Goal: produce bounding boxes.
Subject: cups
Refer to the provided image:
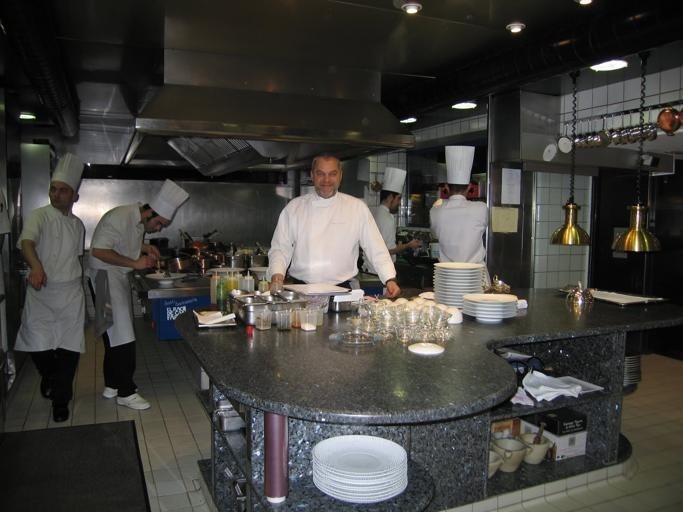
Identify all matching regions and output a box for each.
[522,433,553,465]
[345,298,452,344]
[490,437,532,473]
[487,450,505,479]
[254,298,327,332]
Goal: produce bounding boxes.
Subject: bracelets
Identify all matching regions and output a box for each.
[387,278,400,284]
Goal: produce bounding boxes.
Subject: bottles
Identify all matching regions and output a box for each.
[258,276,268,293]
[209,269,254,311]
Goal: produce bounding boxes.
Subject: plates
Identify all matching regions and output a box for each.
[195,310,237,328]
[433,259,485,308]
[312,434,409,503]
[281,284,351,295]
[461,294,519,325]
[145,273,187,284]
[623,348,642,387]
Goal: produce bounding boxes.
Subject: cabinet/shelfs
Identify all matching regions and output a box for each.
[246,408,493,512]
[488,327,634,512]
[208,384,247,512]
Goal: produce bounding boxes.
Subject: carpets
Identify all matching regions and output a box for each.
[0,420,151,512]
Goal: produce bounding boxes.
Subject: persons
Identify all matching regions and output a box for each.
[429,145,492,287]
[17,152,86,423]
[266,150,402,298]
[362,166,423,275]
[85,179,190,410]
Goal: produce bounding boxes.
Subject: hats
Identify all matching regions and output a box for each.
[444,144,476,186]
[148,177,190,222]
[48,151,85,192]
[381,165,408,195]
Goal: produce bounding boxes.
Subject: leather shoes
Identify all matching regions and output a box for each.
[40,375,56,399]
[52,403,69,421]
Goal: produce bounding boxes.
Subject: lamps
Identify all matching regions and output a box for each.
[610,0,659,251]
[549,0,593,247]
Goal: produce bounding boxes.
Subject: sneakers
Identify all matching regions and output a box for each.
[116,391,152,410]
[102,386,138,399]
